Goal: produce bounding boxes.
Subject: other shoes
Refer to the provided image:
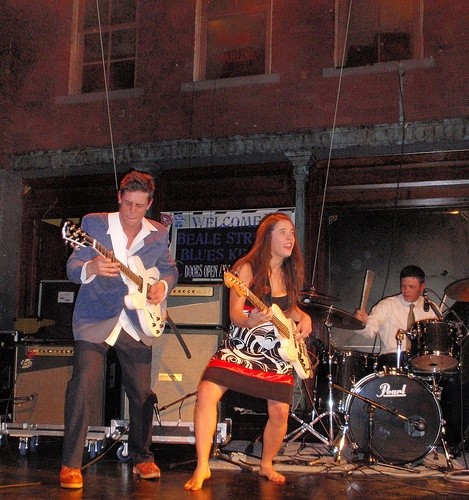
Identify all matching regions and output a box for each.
[59,465,84,488]
[133,462,161,478]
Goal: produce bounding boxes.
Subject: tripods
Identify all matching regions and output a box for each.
[283,321,344,456]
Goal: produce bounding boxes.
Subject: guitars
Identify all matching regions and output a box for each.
[223,262,312,381]
[61,221,168,339]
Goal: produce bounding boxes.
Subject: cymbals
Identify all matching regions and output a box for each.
[299,290,341,302]
[299,302,366,330]
[446,279,469,302]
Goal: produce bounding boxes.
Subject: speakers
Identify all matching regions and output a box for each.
[11,339,105,434]
[120,328,226,427]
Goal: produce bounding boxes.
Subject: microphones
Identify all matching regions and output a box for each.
[414,419,428,431]
[422,290,429,312]
[150,393,162,424]
[27,391,37,402]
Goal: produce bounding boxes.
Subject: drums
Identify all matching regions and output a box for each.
[405,318,459,371]
[314,349,378,411]
[343,370,443,464]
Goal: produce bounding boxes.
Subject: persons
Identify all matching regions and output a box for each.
[353,265,443,372]
[184,213,313,492]
[58,171,179,489]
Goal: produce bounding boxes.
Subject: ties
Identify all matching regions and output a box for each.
[406,304,415,351]
